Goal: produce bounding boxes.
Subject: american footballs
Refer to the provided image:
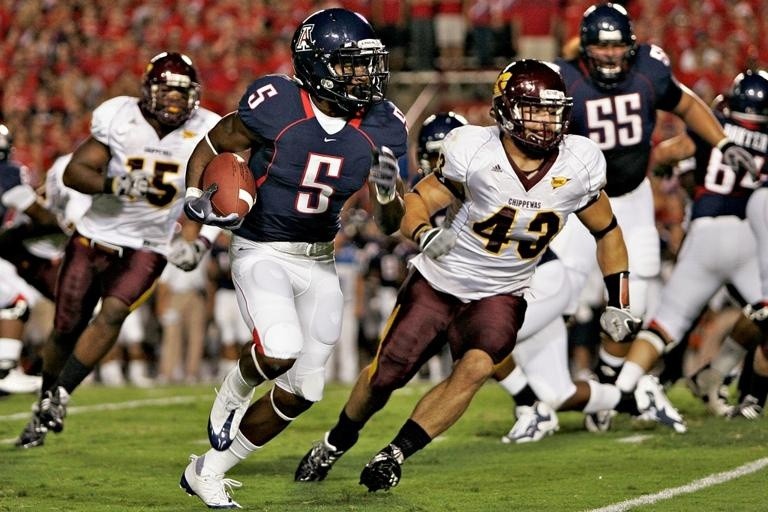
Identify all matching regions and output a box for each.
[203,152,256,222]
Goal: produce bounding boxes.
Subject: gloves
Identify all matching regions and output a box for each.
[101,169,152,199]
[601,308,644,344]
[368,144,400,205]
[716,139,759,184]
[419,227,459,261]
[166,240,206,272]
[183,183,245,231]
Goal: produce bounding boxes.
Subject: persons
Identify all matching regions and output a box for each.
[179,7,407,507]
[340,1,768,258]
[324,229,365,387]
[582,69,768,434]
[15,52,222,446]
[550,0,759,386]
[0,0,319,392]
[293,57,642,492]
[415,111,687,442]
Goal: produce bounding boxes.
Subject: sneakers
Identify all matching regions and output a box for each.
[358,449,400,492]
[15,422,47,449]
[501,370,763,442]
[180,456,242,509]
[208,372,256,452]
[294,428,358,482]
[32,386,67,433]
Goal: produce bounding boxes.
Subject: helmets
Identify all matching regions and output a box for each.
[290,9,390,119]
[139,51,201,127]
[728,69,768,123]
[416,113,469,181]
[581,3,636,91]
[492,59,574,156]
